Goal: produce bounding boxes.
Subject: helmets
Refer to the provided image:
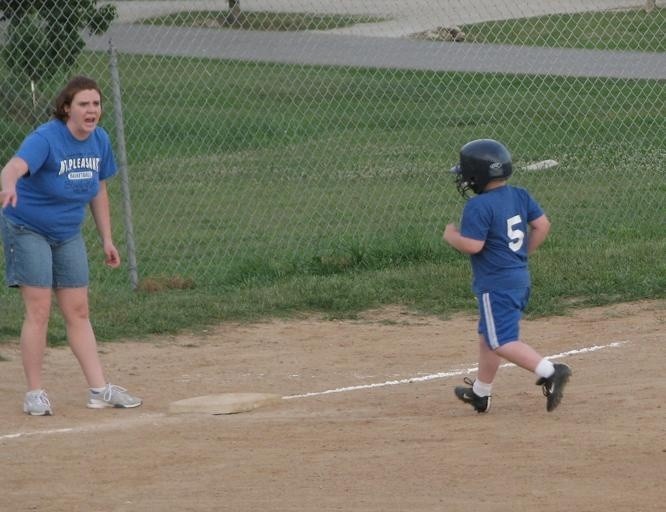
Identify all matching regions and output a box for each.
[450,139,512,199]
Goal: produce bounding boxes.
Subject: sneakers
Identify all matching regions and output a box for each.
[537,363,571,411]
[24,389,52,415]
[455,377,491,413]
[87,384,142,408]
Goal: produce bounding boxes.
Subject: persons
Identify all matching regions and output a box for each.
[0,76,143,417]
[442,138,572,412]
